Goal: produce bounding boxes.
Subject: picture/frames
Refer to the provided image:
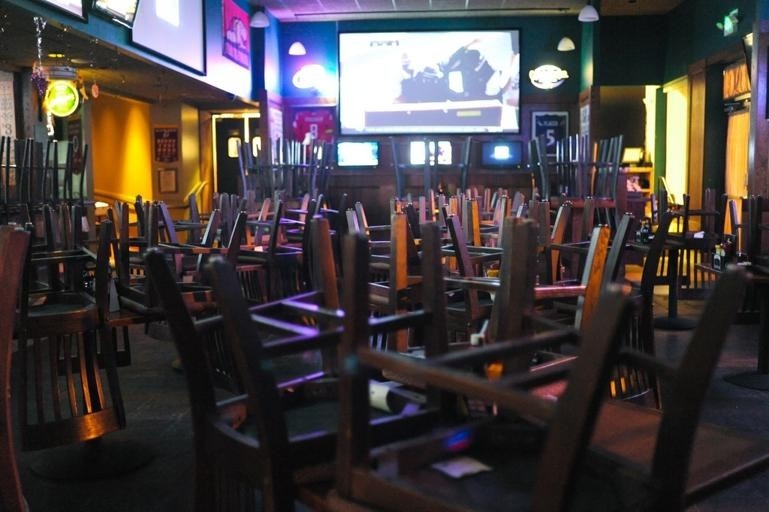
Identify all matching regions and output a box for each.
[157,167,178,193]
[529,109,571,159]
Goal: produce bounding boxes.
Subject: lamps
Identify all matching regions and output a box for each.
[249,11,269,29]
[577,0,599,23]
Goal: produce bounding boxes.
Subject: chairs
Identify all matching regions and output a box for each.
[1,135,768,509]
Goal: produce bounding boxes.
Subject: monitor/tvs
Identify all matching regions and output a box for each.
[480,141,523,170]
[43,0,89,23]
[91,0,139,29]
[336,141,380,169]
[408,140,455,168]
